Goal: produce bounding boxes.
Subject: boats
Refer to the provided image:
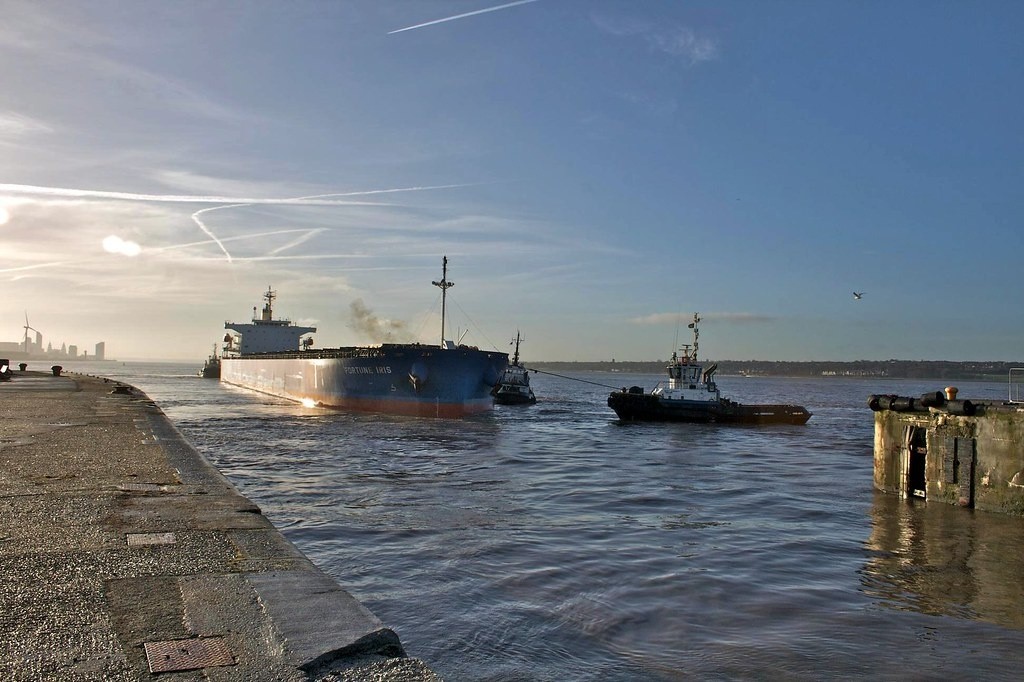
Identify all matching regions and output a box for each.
[608,312,812,425]
[494,330,537,406]
[221,254,512,421]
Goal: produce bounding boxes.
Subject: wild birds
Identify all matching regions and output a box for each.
[852,291,867,301]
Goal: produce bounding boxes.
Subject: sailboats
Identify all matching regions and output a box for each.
[199,342,220,378]
